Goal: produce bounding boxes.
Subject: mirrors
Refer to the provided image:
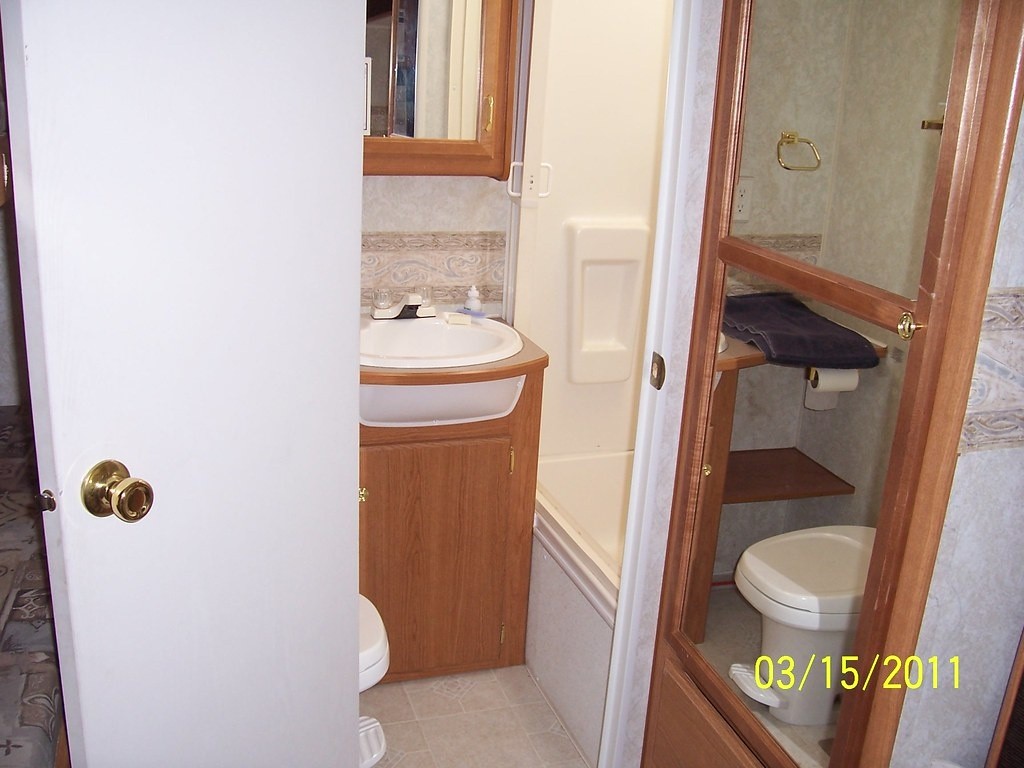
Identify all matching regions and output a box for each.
[666,0,996,768]
[364,0,513,182]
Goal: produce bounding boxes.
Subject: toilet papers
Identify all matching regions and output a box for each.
[803,367,859,411]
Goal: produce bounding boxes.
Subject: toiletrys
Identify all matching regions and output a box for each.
[464,284,482,312]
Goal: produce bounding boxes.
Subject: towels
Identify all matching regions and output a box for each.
[721,291,880,370]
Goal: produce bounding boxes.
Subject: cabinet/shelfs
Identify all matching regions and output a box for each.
[683,322,889,645]
[358,438,514,684]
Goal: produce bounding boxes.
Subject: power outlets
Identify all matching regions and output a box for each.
[731,175,754,223]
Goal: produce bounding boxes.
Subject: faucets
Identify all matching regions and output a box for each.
[369,283,437,320]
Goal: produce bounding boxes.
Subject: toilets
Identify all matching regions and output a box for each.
[728,524,877,724]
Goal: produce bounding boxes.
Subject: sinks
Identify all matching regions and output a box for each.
[359,311,524,369]
